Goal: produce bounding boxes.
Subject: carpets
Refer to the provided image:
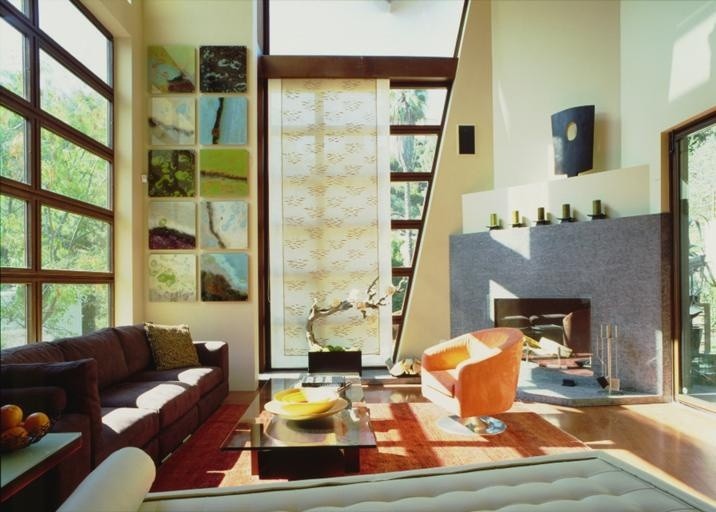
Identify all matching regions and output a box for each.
[148,386,590,493]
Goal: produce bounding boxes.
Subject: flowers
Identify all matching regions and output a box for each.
[307,275,406,352]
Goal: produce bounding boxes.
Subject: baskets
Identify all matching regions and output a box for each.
[0,418,57,451]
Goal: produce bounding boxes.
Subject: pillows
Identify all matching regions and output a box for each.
[144,322,202,371]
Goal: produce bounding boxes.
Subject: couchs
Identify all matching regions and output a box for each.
[0,322,229,511]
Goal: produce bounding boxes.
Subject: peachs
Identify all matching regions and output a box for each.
[1,405,49,440]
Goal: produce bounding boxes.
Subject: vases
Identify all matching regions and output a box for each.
[308,351,362,377]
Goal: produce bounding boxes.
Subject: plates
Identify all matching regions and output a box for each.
[263,396,348,422]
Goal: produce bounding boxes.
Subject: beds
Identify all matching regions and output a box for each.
[57,448,715,511]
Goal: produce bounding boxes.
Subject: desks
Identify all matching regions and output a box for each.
[0,430,83,505]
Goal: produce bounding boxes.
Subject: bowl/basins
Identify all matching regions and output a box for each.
[273,387,340,416]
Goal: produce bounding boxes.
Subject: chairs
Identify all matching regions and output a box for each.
[420,326,524,436]
